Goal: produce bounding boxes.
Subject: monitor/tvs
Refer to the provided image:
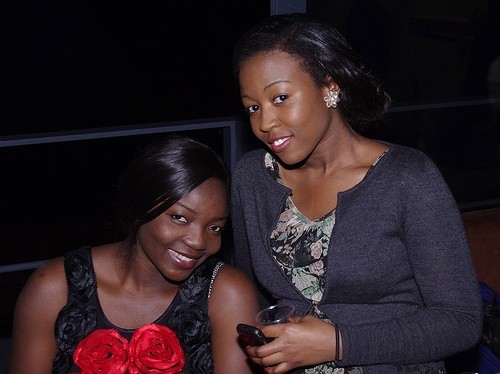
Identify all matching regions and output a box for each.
[351,97,500,212]
[0,117,240,286]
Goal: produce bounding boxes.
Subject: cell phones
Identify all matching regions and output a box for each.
[236,324,268,347]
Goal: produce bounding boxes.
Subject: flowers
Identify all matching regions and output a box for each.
[73,324,185,374]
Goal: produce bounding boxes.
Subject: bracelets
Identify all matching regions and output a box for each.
[336,328,339,360]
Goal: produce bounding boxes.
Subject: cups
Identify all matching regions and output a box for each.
[255,303,297,325]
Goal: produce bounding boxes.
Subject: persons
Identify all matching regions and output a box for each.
[229,12,481,374]
[8,136,260,374]
